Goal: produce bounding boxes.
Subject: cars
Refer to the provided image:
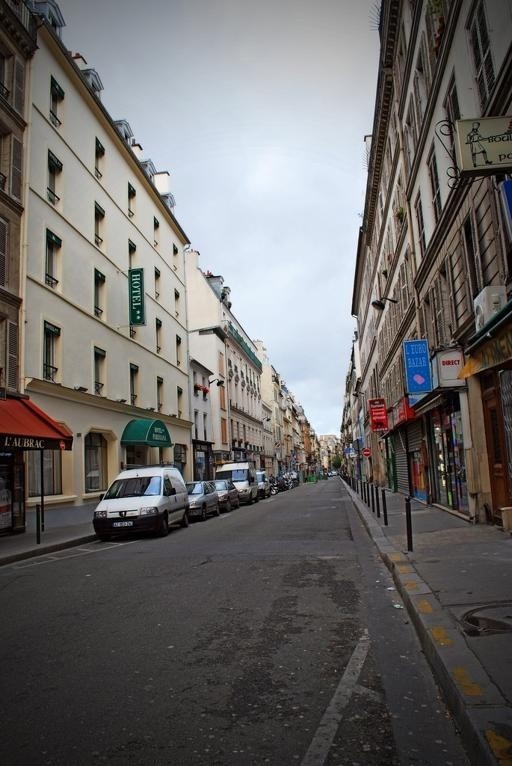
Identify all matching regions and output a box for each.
[185,479,240,522]
[290,471,302,486]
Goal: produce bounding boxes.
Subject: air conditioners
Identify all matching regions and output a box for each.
[472,284,508,333]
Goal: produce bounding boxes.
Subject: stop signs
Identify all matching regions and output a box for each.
[362,448,372,458]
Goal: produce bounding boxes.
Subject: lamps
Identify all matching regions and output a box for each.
[371,296,398,311]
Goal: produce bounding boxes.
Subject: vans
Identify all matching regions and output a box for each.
[215,461,271,504]
[93,466,190,541]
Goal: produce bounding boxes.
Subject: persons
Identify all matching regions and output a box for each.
[311,459,317,474]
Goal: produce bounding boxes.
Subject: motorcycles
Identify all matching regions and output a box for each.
[271,473,293,494]
